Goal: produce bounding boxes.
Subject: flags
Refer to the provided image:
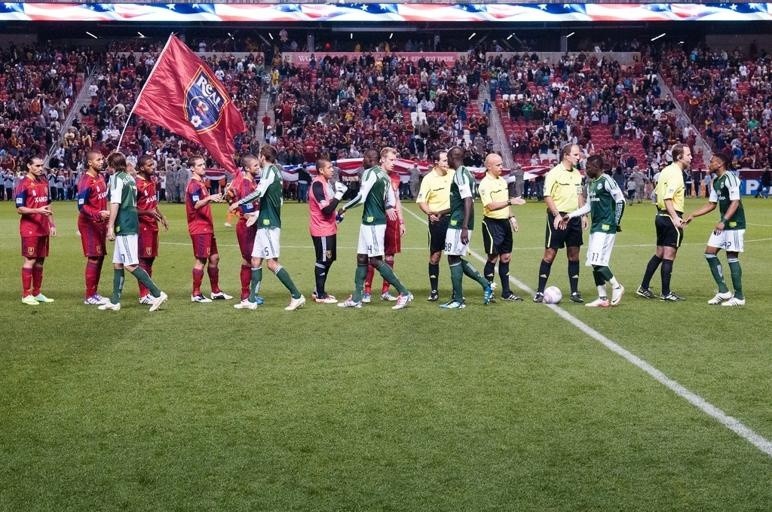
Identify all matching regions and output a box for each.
[116,32,249,174]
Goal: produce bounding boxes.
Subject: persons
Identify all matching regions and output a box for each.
[43,167,82,199]
[683,47,745,153]
[236,155,265,306]
[635,143,685,302]
[229,143,306,312]
[1,41,14,147]
[250,29,306,140]
[56,39,115,150]
[415,150,456,303]
[591,46,634,154]
[59,150,75,200]
[131,155,168,305]
[495,32,532,150]
[607,157,665,202]
[534,44,591,142]
[15,155,59,305]
[635,44,683,143]
[187,155,233,304]
[359,149,406,302]
[532,143,589,302]
[0,150,14,199]
[559,155,626,308]
[746,46,772,169]
[336,148,414,311]
[309,159,345,305]
[479,153,528,304]
[307,30,333,158]
[14,46,56,154]
[685,155,745,307]
[76,151,110,305]
[98,152,168,311]
[335,36,493,148]
[440,145,497,309]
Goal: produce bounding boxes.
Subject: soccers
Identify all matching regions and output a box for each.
[543,286,562,304]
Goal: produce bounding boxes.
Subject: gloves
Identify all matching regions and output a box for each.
[334,209,345,226]
[332,179,348,201]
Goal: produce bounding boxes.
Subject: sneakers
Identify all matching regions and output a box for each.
[148,290,168,312]
[315,296,338,304]
[390,291,415,311]
[35,292,54,302]
[312,292,335,300]
[635,284,658,300]
[337,294,365,309]
[21,293,40,305]
[84,294,109,304]
[610,283,625,306]
[380,291,398,301]
[361,293,372,304]
[482,281,497,306]
[439,297,467,311]
[190,293,213,303]
[500,291,523,302]
[584,297,609,308]
[659,291,686,302]
[706,290,734,305]
[138,293,156,305]
[426,289,439,302]
[233,297,259,312]
[569,292,584,304]
[256,296,264,305]
[720,295,747,308]
[283,293,306,312]
[210,291,234,300]
[97,300,121,311]
[533,290,544,303]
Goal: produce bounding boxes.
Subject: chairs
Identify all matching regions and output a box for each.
[82,97,135,152]
[465,101,481,120]
[403,111,441,130]
[588,125,646,169]
[660,64,689,103]
[528,77,562,92]
[736,80,751,95]
[0,65,82,112]
[495,94,542,136]
[514,153,550,166]
[311,67,339,91]
